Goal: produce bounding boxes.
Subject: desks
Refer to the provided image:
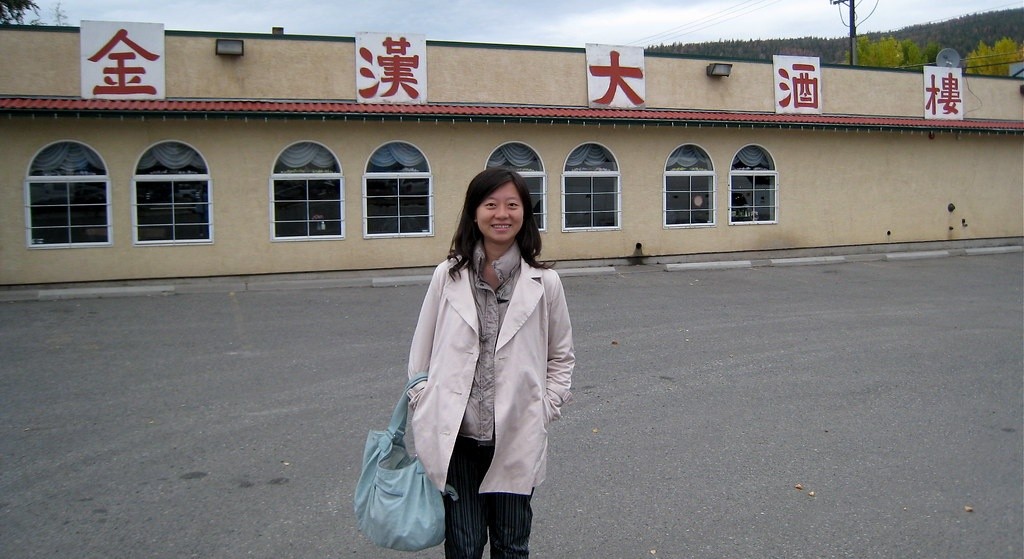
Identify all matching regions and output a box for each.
[734,215,760,220]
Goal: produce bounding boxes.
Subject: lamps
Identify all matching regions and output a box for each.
[706,63,732,77]
[272,27,283,35]
[215,38,244,56]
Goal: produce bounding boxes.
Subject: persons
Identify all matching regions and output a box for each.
[408,167,576,559]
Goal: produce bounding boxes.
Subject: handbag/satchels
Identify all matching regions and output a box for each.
[353,371,458,551]
